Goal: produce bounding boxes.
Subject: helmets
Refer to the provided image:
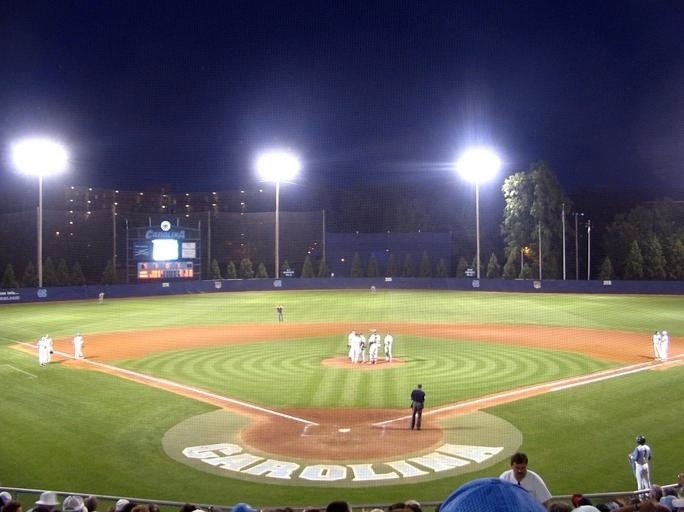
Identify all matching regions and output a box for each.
[636,435,645,444]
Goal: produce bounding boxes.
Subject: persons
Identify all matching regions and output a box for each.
[659,331,668,359]
[384,333,393,363]
[439,474,683,512]
[98,292,104,304]
[500,452,551,508]
[652,331,661,360]
[276,305,284,323]
[72,333,84,359]
[2,491,421,512]
[410,384,425,430]
[348,328,381,365]
[628,435,652,502]
[38,334,53,365]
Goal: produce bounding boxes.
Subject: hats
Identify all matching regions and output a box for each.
[116,500,129,510]
[440,479,547,512]
[0,492,12,505]
[231,503,257,512]
[63,496,84,511]
[571,494,602,512]
[35,491,60,505]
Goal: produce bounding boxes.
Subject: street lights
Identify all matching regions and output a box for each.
[252,148,301,280]
[455,148,504,279]
[11,137,68,289]
